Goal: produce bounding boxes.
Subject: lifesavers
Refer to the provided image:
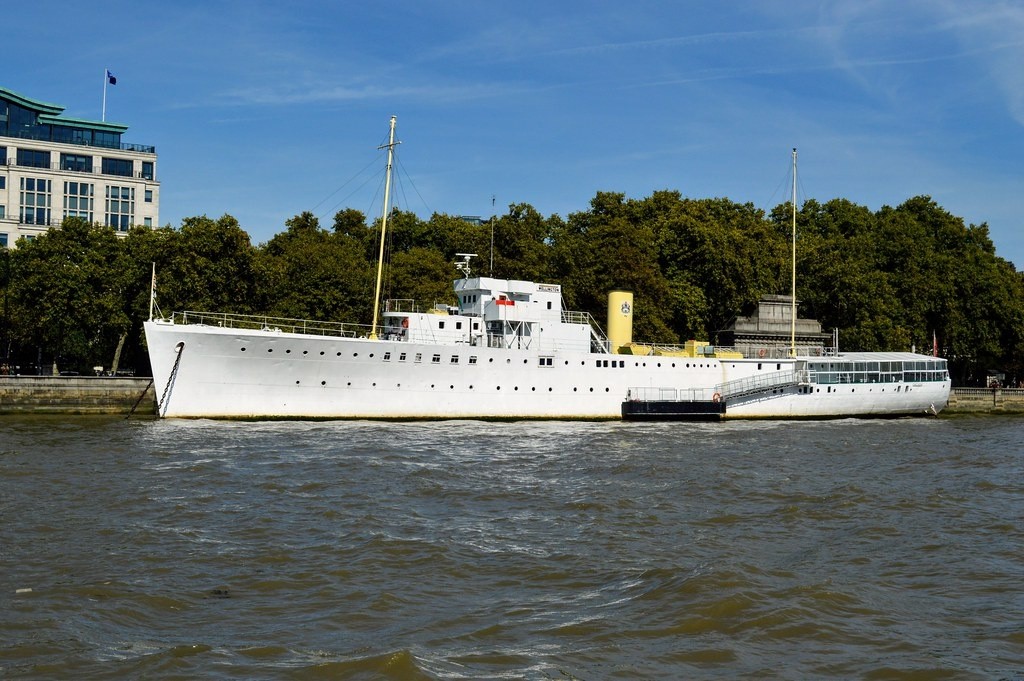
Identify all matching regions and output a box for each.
[401,319,408,328]
[758,349,765,357]
[713,393,720,402]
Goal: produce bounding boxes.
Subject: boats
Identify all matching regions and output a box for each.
[143,112,953,421]
